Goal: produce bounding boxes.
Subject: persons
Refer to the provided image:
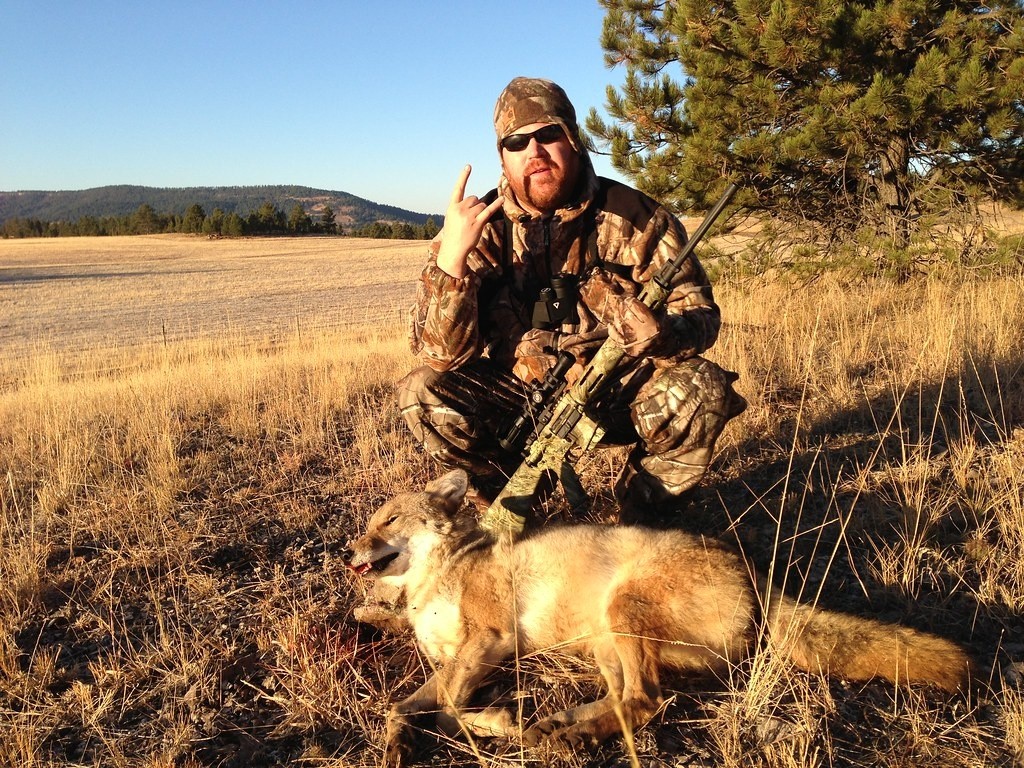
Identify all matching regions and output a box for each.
[395,75,751,534]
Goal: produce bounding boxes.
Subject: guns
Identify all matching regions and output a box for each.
[478,181,741,551]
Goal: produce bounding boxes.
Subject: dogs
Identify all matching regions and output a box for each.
[343,469,976,768]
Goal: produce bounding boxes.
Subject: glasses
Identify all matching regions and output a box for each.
[499,123,565,152]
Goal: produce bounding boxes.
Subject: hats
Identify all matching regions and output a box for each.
[493,76,582,155]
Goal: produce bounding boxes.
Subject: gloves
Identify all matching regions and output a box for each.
[606,297,678,359]
